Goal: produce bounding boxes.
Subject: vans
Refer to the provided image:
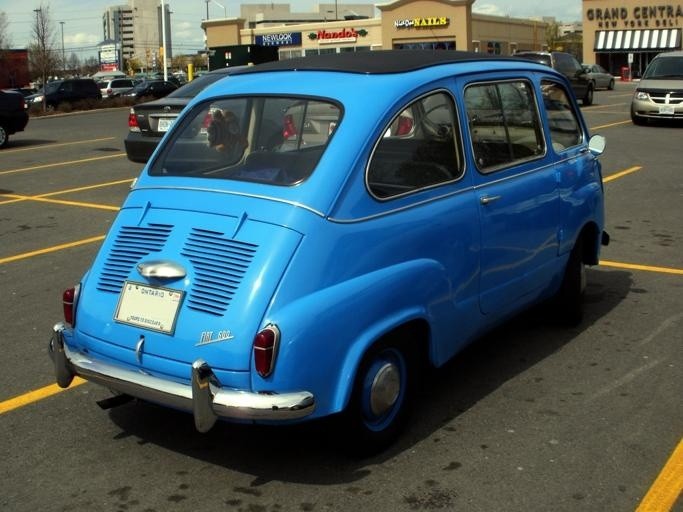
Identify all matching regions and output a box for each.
[48,56,609,448]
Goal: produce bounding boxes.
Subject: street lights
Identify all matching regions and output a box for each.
[59,20,65,67]
[33,9,40,47]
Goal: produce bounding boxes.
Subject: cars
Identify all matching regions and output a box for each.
[630,52,682,126]
[273,84,537,152]
[0,60,253,164]
[514,52,614,106]
